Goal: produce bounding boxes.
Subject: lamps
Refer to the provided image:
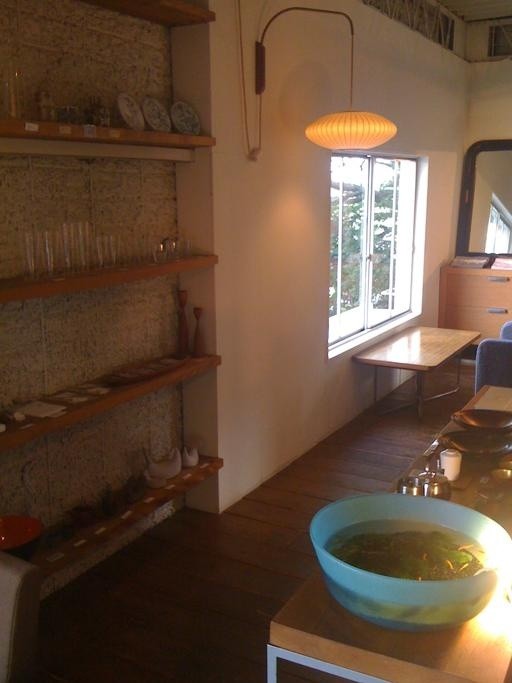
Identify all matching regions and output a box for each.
[252,6,399,154]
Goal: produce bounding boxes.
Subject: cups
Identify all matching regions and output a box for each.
[310,490,511,629]
[397,476,421,495]
[23,220,192,278]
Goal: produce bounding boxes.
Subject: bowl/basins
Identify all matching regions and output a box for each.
[450,407,512,433]
[1,514,44,559]
[437,433,512,458]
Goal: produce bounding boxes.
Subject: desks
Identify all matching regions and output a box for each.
[352,324,482,422]
[438,265,511,348]
[263,383,512,683]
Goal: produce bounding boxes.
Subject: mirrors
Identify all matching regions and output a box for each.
[456,136,512,257]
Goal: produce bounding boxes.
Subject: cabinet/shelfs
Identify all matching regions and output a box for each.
[0,1,226,610]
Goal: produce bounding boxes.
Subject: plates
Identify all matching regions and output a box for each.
[116,93,201,135]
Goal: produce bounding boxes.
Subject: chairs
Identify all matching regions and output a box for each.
[475,335,512,397]
[500,318,512,341]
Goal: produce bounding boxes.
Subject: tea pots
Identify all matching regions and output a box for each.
[416,471,451,503]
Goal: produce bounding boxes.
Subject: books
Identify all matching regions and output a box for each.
[10,398,67,419]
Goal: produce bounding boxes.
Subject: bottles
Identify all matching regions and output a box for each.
[36,91,113,123]
[440,448,462,481]
